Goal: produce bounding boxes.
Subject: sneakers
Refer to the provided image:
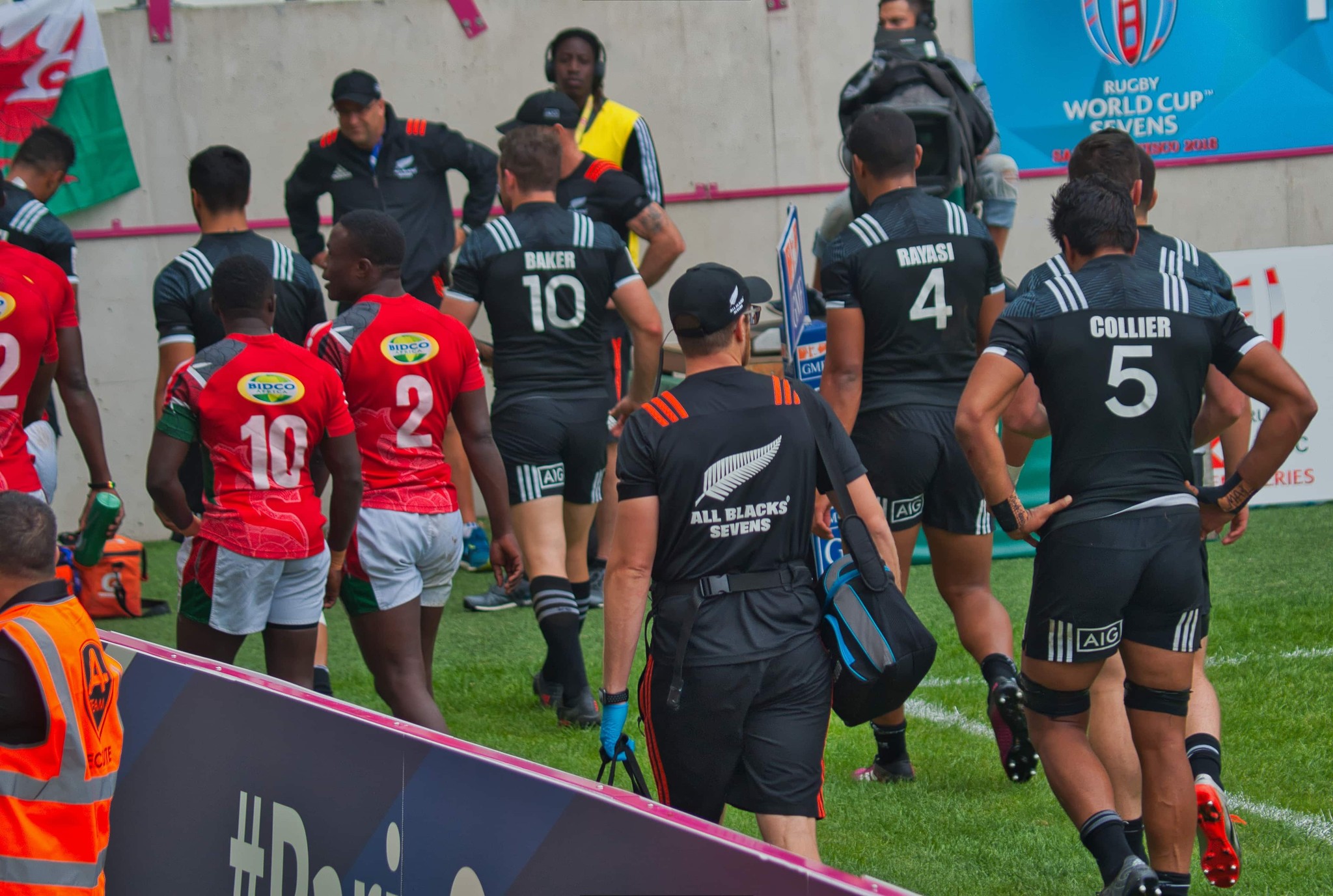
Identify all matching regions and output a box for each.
[311,666,333,697]
[554,689,598,729]
[1192,775,1241,888]
[585,565,606,611]
[852,759,914,786]
[987,677,1039,786]
[464,574,534,612]
[458,522,495,571]
[530,670,565,708]
[1095,855,1162,896]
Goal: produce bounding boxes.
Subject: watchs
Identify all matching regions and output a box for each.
[598,686,629,706]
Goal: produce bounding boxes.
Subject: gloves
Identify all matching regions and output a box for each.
[600,704,637,761]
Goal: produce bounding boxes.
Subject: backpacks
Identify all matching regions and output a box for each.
[849,53,990,232]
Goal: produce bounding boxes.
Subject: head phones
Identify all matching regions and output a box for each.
[918,0,937,31]
[545,27,607,82]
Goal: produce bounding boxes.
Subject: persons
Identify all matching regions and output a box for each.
[766,1,1023,319]
[601,260,902,866]
[285,68,503,574]
[1,488,124,896]
[985,146,1250,548]
[438,125,662,731]
[0,169,124,567]
[145,256,361,692]
[544,26,667,279]
[0,123,82,506]
[302,209,526,735]
[154,146,332,702]
[953,173,1319,896]
[809,105,1041,786]
[1001,128,1248,889]
[461,90,687,614]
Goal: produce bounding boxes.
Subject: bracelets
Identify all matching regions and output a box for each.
[88,481,115,489]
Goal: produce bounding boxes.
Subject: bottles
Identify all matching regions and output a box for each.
[74,492,121,567]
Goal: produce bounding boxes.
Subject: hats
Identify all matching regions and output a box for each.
[670,263,774,335]
[327,68,380,109]
[495,90,581,136]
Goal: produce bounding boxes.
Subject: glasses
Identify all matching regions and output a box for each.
[740,305,760,326]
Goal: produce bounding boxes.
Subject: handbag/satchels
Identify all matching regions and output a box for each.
[57,526,151,623]
[789,512,936,733]
